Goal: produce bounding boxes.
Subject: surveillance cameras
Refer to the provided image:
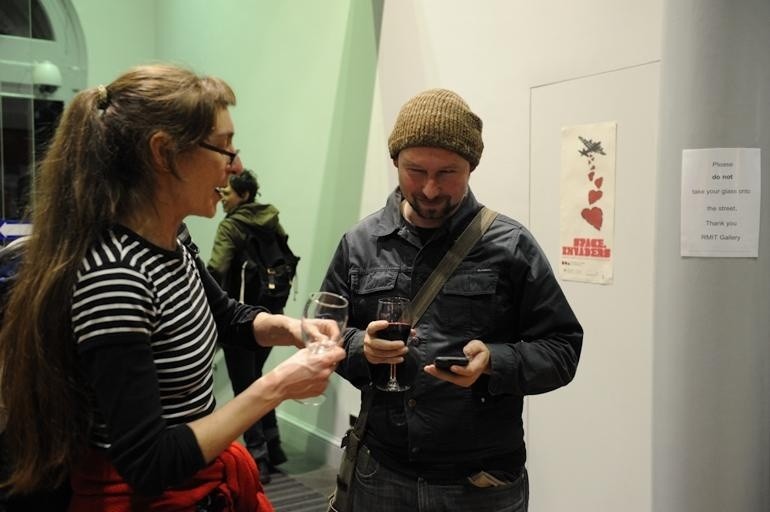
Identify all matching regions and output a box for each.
[34,60,62,96]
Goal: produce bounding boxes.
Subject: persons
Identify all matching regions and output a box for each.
[205,168,304,486]
[312,87,587,512]
[0,63,349,509]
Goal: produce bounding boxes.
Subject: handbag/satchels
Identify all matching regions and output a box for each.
[326,428,359,510]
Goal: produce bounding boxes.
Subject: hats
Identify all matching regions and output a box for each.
[389,89,483,171]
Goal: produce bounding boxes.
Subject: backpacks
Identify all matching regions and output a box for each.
[229,227,301,314]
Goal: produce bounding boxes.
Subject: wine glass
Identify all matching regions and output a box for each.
[375,294,413,392]
[290,292,349,408]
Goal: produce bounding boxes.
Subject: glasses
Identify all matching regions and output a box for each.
[195,141,241,164]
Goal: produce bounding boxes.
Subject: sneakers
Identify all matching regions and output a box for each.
[256,444,287,484]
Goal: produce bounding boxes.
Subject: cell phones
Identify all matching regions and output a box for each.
[435,356,469,374]
